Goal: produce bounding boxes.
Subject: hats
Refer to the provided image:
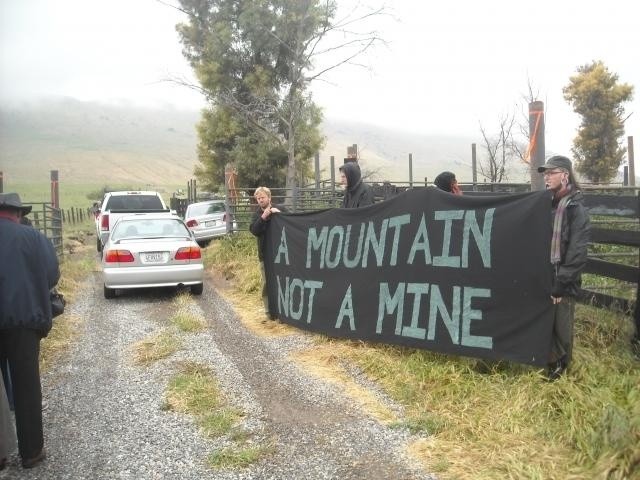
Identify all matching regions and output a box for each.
[1,193,33,218]
[537,155,572,173]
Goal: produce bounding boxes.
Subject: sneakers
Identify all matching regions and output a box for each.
[22,447,47,469]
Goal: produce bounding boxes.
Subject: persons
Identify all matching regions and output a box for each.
[339,161,376,210]
[534,154,591,385]
[249,186,289,324]
[0,192,62,469]
[432,170,463,195]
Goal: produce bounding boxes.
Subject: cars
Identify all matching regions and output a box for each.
[94,187,238,301]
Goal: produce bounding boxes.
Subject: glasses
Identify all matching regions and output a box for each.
[542,171,562,177]
